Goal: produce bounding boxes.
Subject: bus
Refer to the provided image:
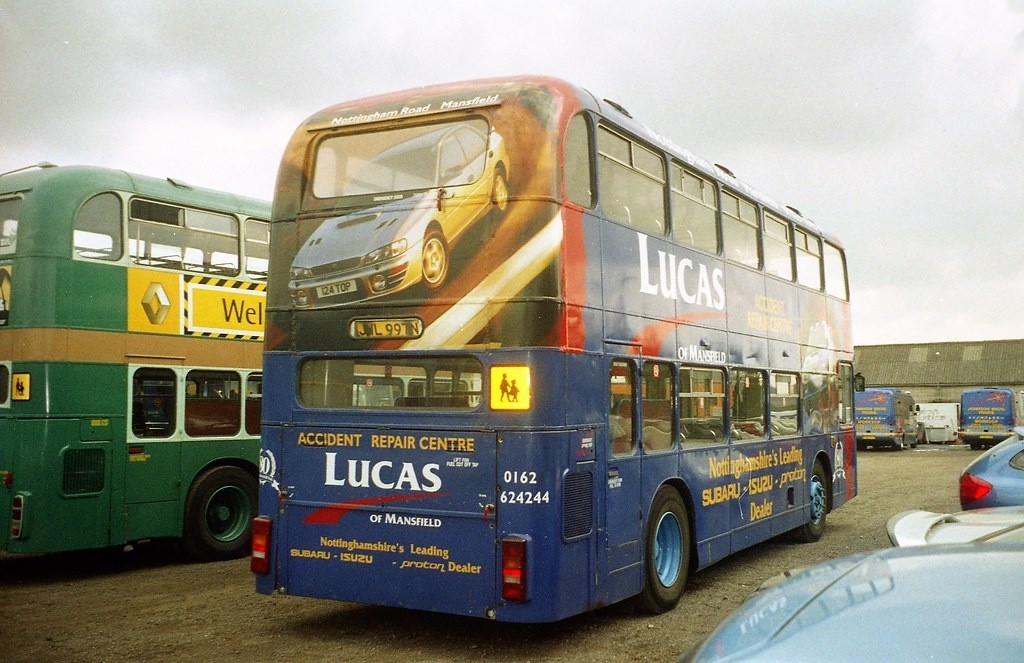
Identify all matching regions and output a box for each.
[0,161,485,560]
[957,386,1018,450]
[250,72,870,623]
[854,387,921,450]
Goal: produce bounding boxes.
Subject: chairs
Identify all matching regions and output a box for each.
[609,417,790,456]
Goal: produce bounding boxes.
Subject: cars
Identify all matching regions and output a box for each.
[679,543,1024,663]
[957,427,1024,510]
[286,125,511,321]
[885,504,1024,547]
